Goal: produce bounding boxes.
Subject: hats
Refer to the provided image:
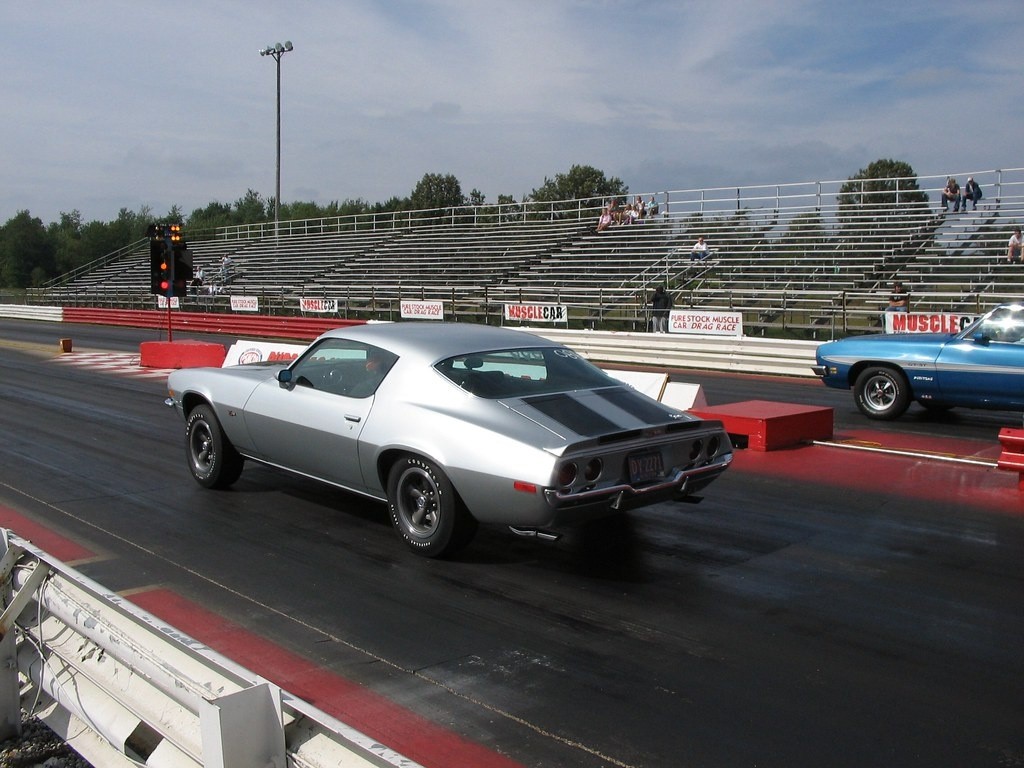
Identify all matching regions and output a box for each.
[968,177,973,181]
[894,282,902,286]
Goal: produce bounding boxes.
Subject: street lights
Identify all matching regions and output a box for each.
[259,40,294,242]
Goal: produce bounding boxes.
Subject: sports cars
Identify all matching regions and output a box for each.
[809,296,1024,422]
[165,318,738,563]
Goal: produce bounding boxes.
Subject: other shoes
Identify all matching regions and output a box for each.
[943,207,948,212]
[961,206,965,211]
[953,208,958,212]
[973,206,976,210]
[1007,259,1012,264]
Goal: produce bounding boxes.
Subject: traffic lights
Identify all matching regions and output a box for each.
[150,224,193,343]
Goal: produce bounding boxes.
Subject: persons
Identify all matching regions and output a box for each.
[961,177,982,211]
[1007,229,1024,264]
[347,351,387,398]
[590,195,659,236]
[190,254,232,302]
[880,282,909,326]
[651,286,672,333]
[942,178,961,212]
[691,237,709,261]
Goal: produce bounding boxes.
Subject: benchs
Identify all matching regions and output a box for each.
[43,197,1024,340]
